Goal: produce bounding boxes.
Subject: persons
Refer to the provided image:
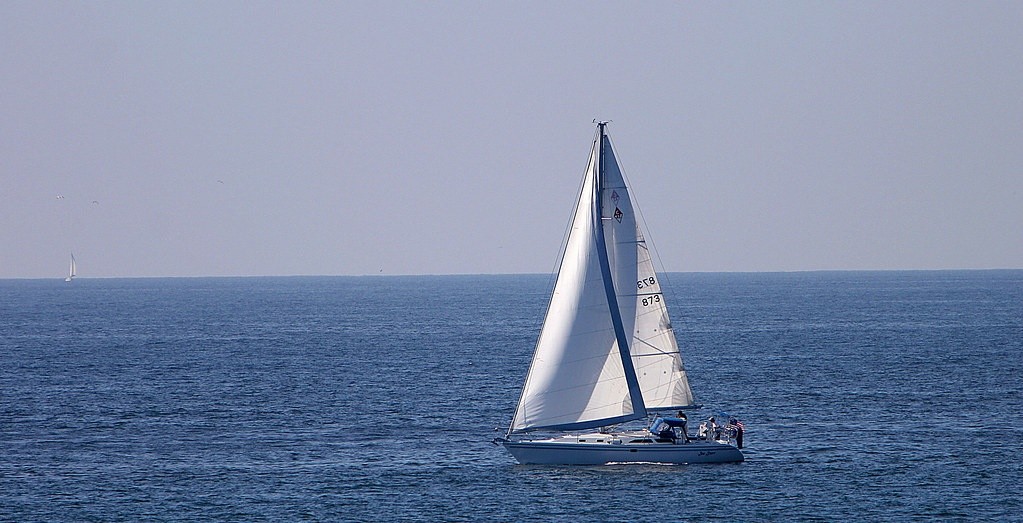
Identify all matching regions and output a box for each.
[708,416,719,432]
[675,411,687,420]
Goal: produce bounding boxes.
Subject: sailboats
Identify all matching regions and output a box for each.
[489,118,745,469]
